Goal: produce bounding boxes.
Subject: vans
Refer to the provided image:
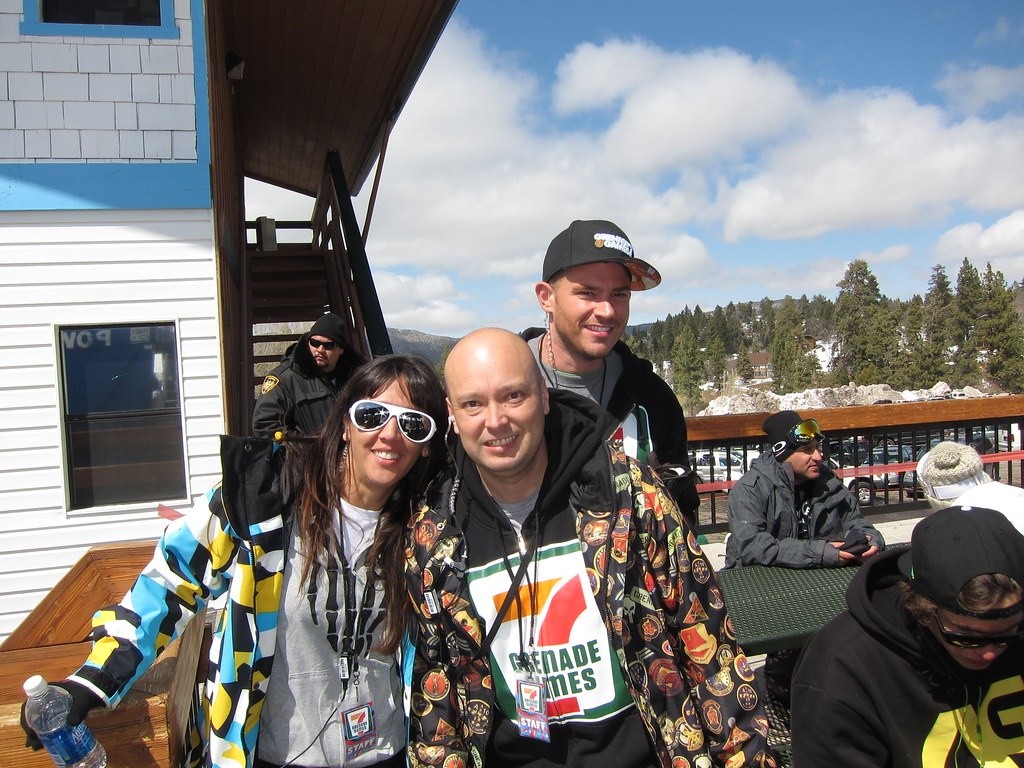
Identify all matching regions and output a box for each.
[997,424,1021,452]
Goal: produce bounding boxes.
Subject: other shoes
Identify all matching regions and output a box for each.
[764,703,791,739]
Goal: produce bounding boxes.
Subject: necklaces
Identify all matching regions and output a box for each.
[539,332,607,406]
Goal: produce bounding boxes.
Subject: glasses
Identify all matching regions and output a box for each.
[791,419,826,443]
[347,399,438,444]
[934,613,1017,647]
[309,337,336,350]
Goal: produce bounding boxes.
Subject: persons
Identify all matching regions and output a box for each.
[725,411,885,569]
[252,312,368,440]
[520,220,700,538]
[20,355,448,768]
[917,441,1024,534]
[400,327,778,768]
[789,506,1024,768]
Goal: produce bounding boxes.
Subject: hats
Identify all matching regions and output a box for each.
[898,506,1024,620]
[763,411,804,461]
[916,441,983,513]
[310,313,347,348]
[543,220,661,290]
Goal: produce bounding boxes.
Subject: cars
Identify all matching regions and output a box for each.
[902,433,997,500]
[688,442,771,494]
[820,453,885,506]
[861,445,912,489]
[860,429,949,446]
[948,426,996,444]
[872,391,993,404]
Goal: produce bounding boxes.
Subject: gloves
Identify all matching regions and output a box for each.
[20,680,107,752]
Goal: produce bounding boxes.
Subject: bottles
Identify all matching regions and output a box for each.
[23,674,107,768]
